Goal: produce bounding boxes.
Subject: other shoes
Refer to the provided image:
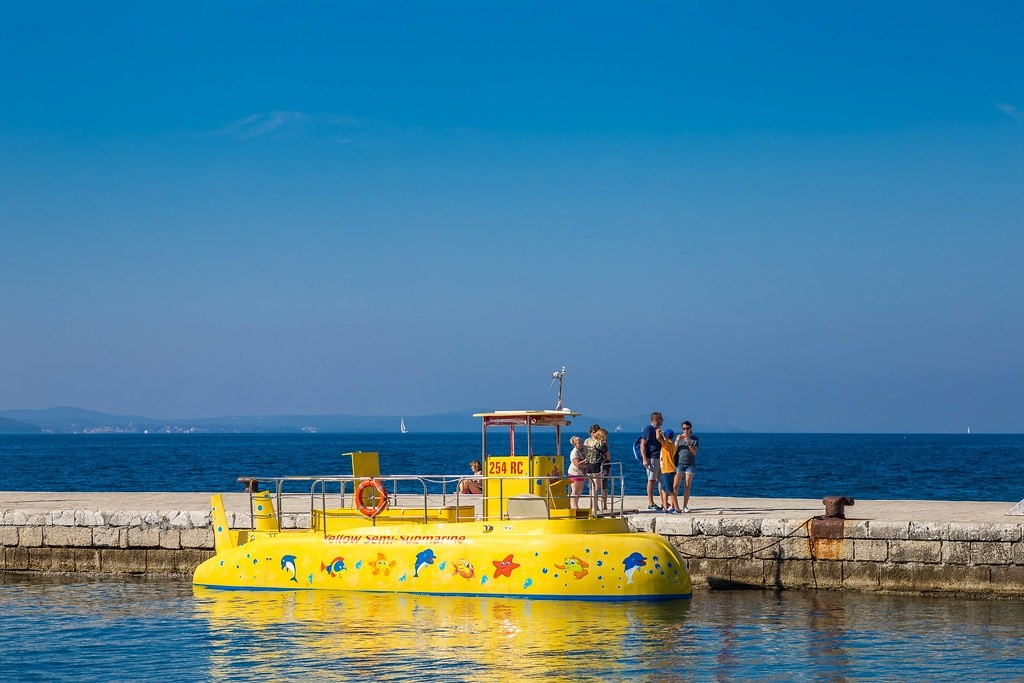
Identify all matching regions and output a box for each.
[654,509,670,515]
[676,510,681,514]
[683,506,691,513]
[667,509,677,514]
[647,504,662,511]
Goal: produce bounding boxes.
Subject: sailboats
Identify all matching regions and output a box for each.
[400,417,408,433]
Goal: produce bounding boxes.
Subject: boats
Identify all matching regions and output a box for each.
[192,364,693,601]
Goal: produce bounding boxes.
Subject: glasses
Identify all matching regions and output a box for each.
[683,428,691,430]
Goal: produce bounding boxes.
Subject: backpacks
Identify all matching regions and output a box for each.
[633,425,657,462]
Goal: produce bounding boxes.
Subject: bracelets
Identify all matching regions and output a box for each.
[687,443,691,445]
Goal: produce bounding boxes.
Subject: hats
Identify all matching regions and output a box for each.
[665,429,674,439]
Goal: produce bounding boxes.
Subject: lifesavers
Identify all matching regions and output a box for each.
[354,479,388,518]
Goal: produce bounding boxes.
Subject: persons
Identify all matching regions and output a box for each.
[667,421,699,513]
[568,436,589,510]
[583,424,610,518]
[655,428,683,515]
[639,412,675,509]
[459,460,482,494]
